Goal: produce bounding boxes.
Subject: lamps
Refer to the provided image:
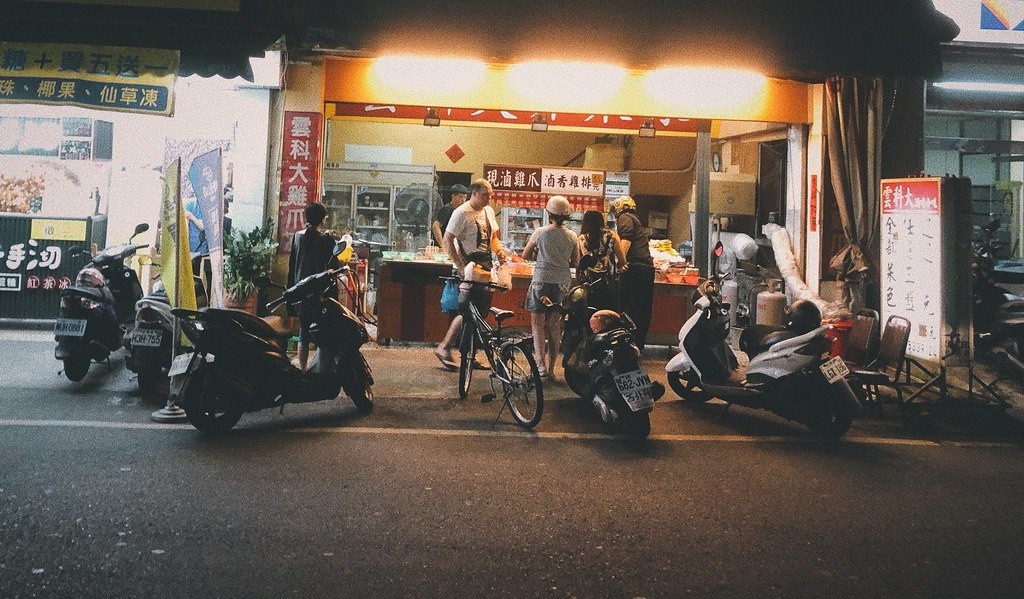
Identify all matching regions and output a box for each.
[531,115,548,132]
[639,118,655,138]
[424,109,440,127]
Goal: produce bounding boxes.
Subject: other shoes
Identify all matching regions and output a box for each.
[548,372,555,379]
[538,370,549,376]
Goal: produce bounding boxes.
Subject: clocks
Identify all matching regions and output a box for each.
[713,153,719,171]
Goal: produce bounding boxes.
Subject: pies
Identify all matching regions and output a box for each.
[648,238,673,254]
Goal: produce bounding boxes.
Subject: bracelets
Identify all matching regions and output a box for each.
[497,249,501,254]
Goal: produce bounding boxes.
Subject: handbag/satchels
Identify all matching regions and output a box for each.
[497,262,512,289]
[440,279,459,311]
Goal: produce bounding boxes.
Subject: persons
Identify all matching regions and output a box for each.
[522,196,581,379]
[611,195,655,350]
[576,210,626,311]
[433,178,507,370]
[287,202,337,370]
[183,197,231,306]
[432,184,468,248]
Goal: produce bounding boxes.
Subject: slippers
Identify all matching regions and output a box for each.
[434,350,458,370]
[473,361,490,370]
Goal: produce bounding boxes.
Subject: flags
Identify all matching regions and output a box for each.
[160,173,198,349]
[188,148,225,308]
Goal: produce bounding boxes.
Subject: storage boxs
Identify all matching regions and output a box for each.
[649,218,668,229]
[692,172,756,214]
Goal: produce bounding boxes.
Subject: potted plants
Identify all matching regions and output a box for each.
[223,216,279,313]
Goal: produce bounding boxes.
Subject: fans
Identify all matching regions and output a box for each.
[394,182,443,252]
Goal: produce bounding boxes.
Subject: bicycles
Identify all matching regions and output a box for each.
[439,274,545,430]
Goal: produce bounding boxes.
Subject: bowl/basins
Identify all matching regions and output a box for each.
[400,252,416,261]
[433,254,448,262]
[382,250,398,260]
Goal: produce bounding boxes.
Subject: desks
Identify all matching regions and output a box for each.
[376,256,699,360]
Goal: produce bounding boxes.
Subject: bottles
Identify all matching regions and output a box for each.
[364,193,370,207]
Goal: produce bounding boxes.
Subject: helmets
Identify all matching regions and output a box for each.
[546,196,571,215]
[614,195,636,216]
[76,268,104,287]
[786,299,822,328]
[590,309,619,333]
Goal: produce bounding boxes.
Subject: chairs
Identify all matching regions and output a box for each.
[832,307,911,427]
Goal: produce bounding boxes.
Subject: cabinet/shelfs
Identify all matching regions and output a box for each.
[321,160,435,289]
[501,205,586,256]
[59,118,112,161]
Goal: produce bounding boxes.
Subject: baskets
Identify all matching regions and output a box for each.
[460,287,494,318]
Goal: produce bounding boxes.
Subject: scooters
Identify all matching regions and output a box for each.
[539,277,666,436]
[123,235,211,407]
[191,276,196,279]
[167,263,375,434]
[971,211,1024,380]
[665,239,868,436]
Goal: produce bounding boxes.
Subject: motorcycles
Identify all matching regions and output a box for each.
[53,221,150,381]
